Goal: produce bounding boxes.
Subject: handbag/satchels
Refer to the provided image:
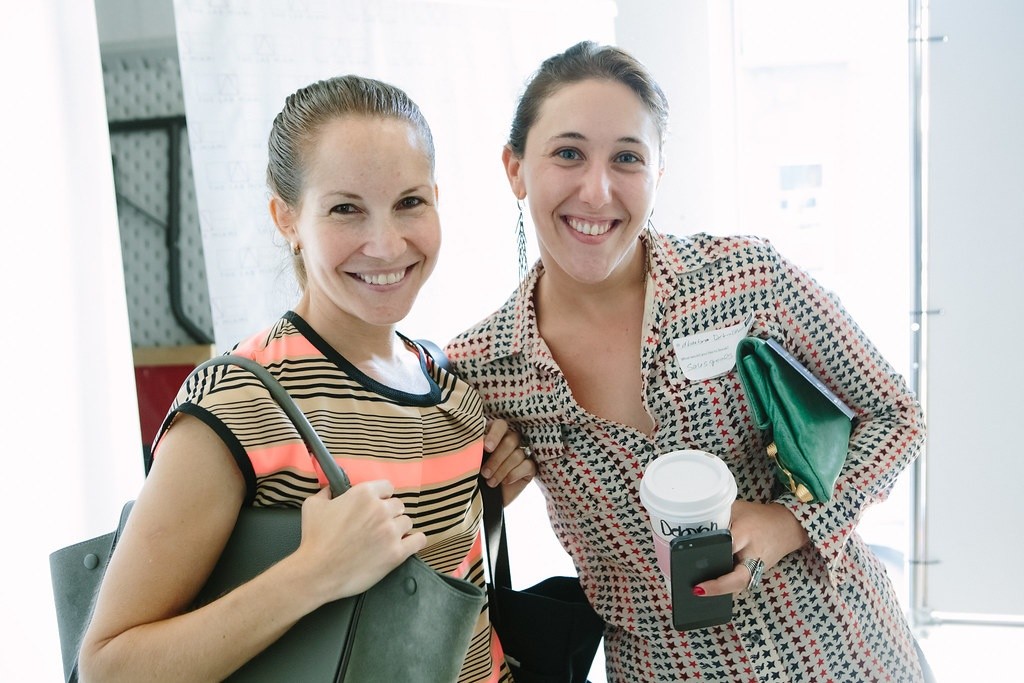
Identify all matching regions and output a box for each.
[736,337,852,505]
[49,357,485,683]
[487,576,606,683]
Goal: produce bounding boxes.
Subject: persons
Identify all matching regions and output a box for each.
[439,40,928,683]
[75,75,537,683]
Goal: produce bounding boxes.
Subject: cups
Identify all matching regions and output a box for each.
[639,449,738,598]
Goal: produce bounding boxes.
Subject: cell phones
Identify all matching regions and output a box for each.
[669,529,732,631]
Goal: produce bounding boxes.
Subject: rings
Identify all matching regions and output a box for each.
[740,556,764,590]
[518,445,532,458]
